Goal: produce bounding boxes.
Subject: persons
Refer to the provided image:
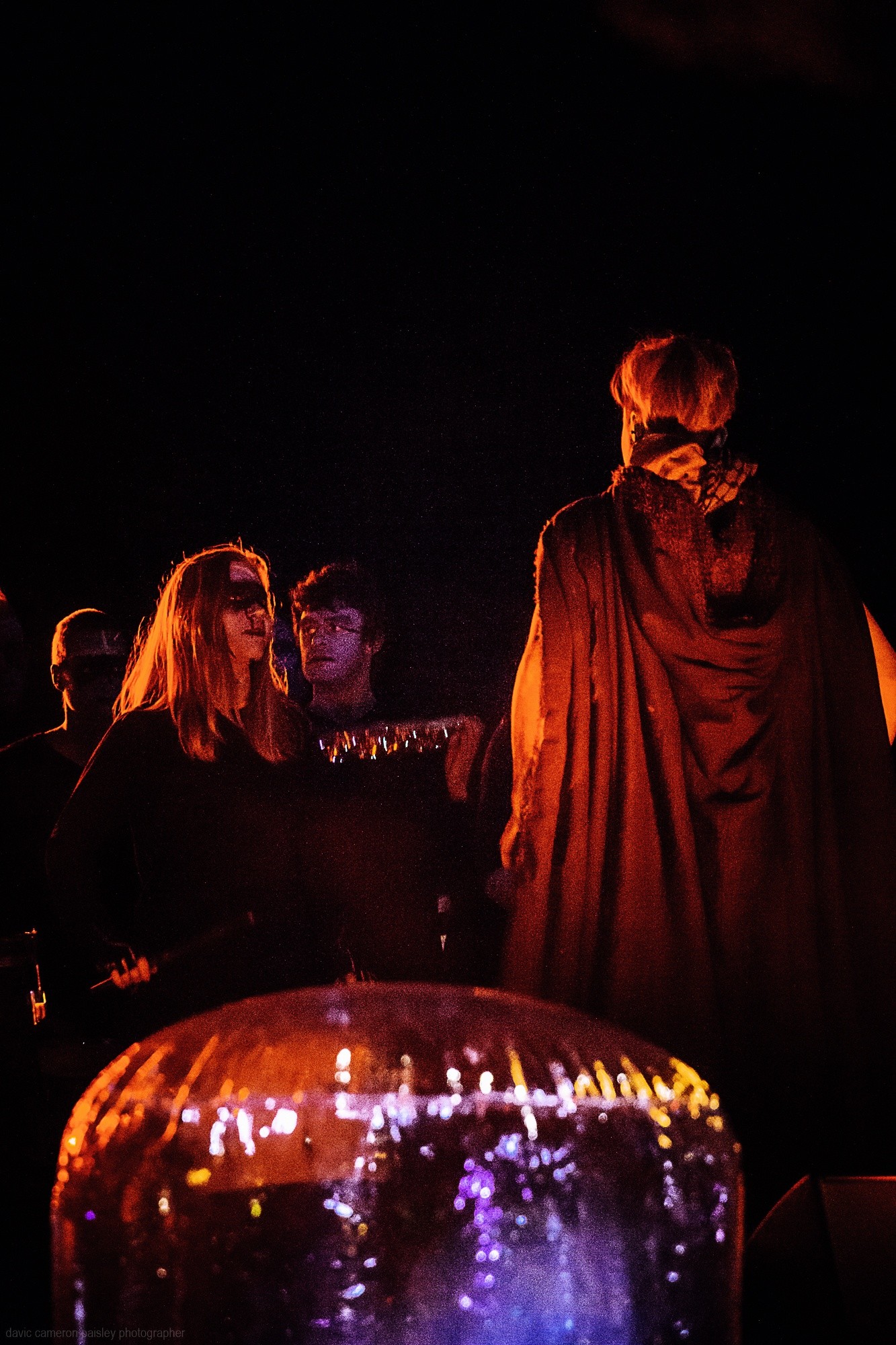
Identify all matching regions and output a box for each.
[36,545,327,1094]
[289,558,482,983]
[499,334,896,1231]
[0,607,131,950]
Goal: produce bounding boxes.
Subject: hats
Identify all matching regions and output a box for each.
[51,608,129,667]
